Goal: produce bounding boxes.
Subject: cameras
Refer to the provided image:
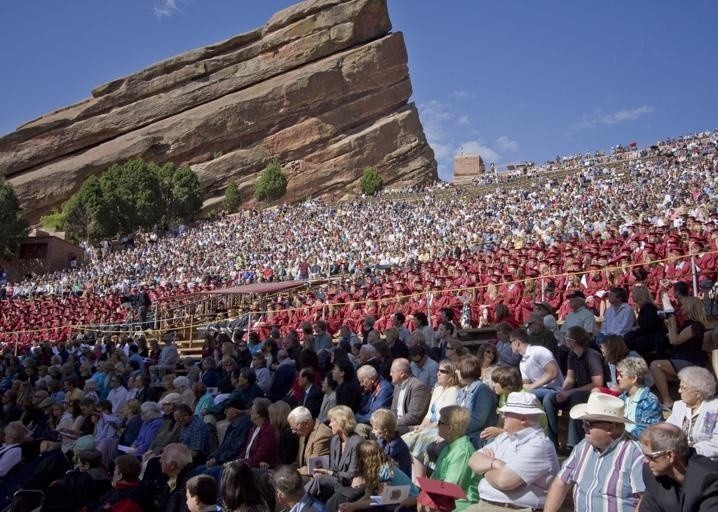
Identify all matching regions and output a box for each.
[657,311,674,319]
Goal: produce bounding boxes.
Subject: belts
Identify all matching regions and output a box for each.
[482,499,543,512]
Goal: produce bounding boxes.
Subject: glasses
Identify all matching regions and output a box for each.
[644,450,666,461]
[583,420,612,429]
[437,368,449,373]
[438,421,449,426]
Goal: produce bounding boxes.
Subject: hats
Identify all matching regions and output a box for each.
[102,360,116,370]
[566,290,586,299]
[498,392,547,417]
[533,301,552,313]
[160,393,182,405]
[74,435,96,450]
[35,430,63,442]
[569,391,635,424]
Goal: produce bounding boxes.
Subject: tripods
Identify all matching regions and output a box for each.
[124,303,140,339]
